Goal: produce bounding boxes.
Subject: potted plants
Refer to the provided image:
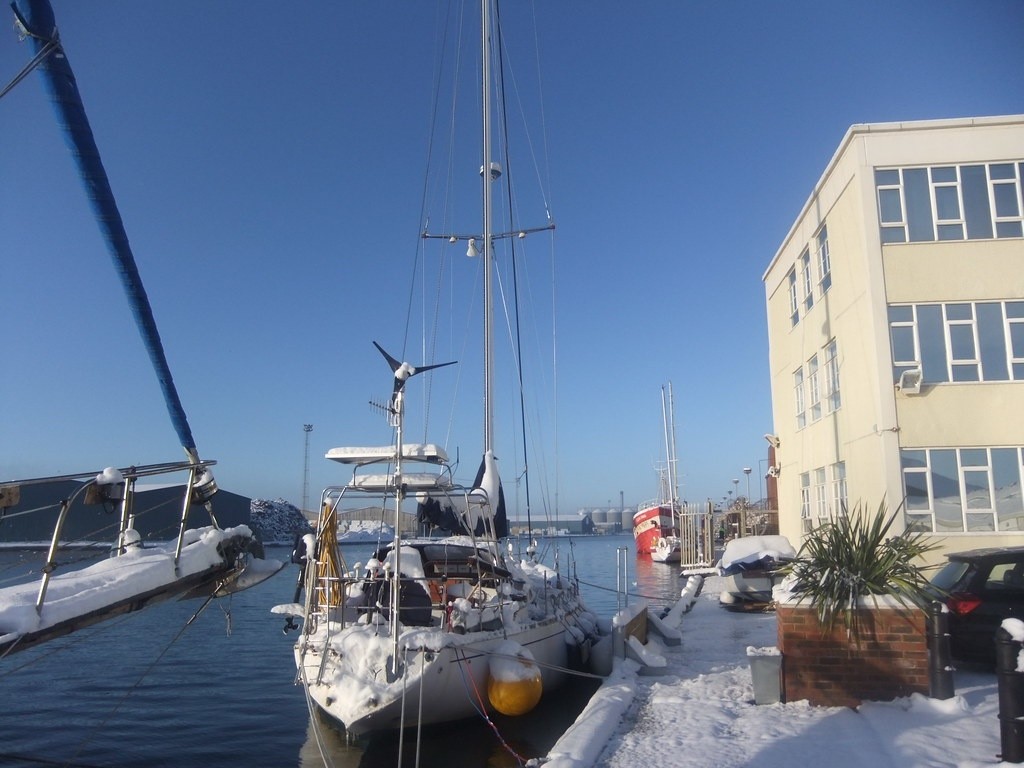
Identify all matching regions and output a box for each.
[771,492,954,708]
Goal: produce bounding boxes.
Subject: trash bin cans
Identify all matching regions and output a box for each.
[748,644,783,705]
[994,619,1024,762]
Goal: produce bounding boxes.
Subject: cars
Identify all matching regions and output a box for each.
[921,545,1024,674]
[720,535,799,613]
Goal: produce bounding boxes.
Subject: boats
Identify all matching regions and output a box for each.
[0,0,257,768]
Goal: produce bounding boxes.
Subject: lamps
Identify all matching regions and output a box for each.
[896,369,922,394]
[762,434,779,448]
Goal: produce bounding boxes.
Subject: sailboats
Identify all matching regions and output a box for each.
[632,379,696,564]
[270,0,605,740]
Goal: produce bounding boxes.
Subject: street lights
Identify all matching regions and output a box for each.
[732,478,739,499]
[299,422,314,517]
[723,496,727,509]
[744,467,751,511]
[728,490,733,506]
[715,504,720,510]
[720,501,724,509]
